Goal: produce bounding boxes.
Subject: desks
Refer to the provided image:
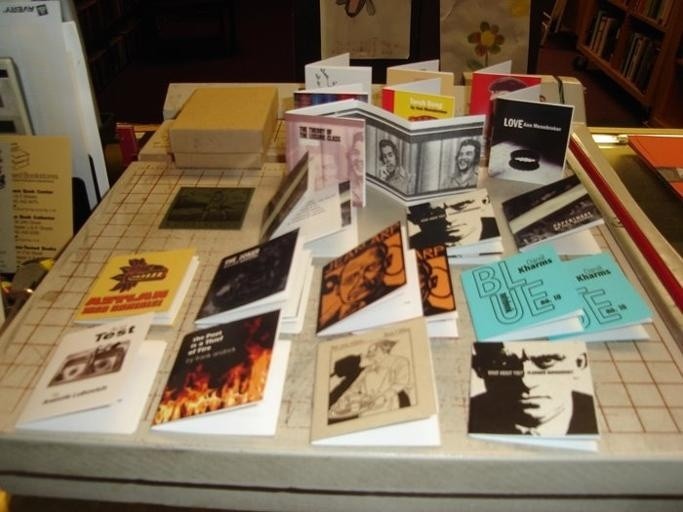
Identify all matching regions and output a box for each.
[1,98,683,512]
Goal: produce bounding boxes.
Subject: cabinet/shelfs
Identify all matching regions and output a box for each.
[575,0,683,128]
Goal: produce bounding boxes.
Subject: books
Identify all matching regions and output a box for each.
[150,309,291,435]
[75,53,655,342]
[468,341,600,450]
[585,1,672,92]
[311,316,440,447]
[15,311,168,437]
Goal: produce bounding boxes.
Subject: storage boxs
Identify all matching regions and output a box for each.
[170,84,281,169]
